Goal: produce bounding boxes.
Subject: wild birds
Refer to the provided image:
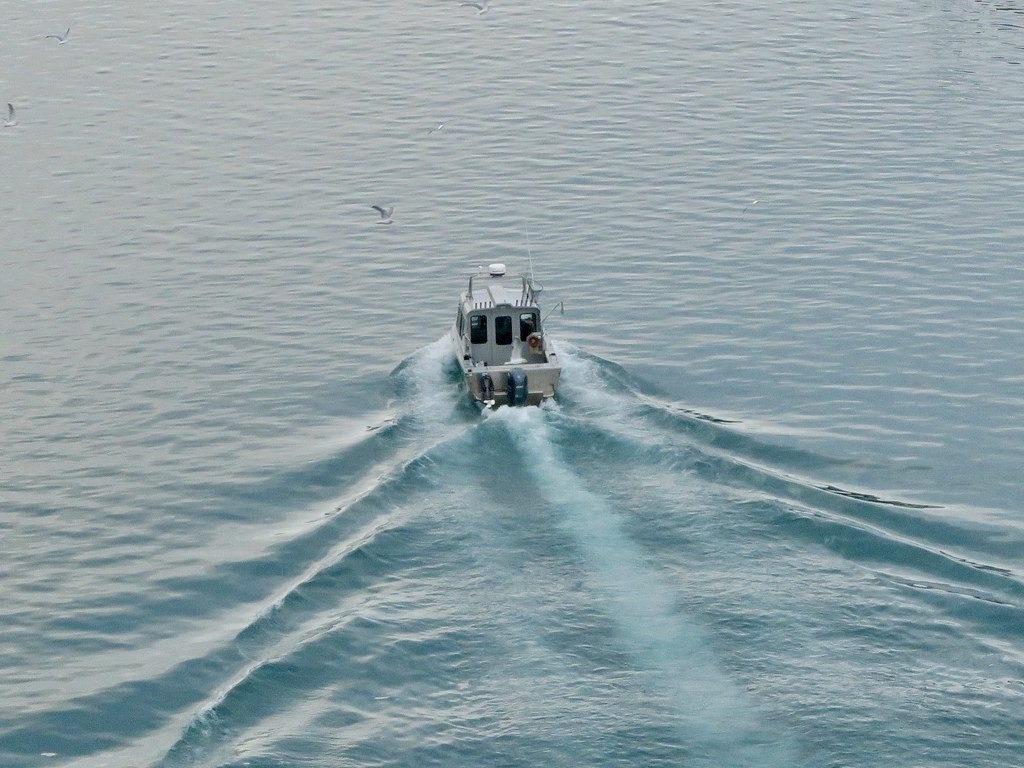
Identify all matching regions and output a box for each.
[44,27,73,45]
[2,103,19,130]
[458,0,496,18]
[368,203,398,226]
[427,122,447,135]
[742,199,769,214]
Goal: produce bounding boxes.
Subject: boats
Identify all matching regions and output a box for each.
[448,263,563,413]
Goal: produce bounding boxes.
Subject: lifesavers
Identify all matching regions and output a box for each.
[528,336,539,348]
